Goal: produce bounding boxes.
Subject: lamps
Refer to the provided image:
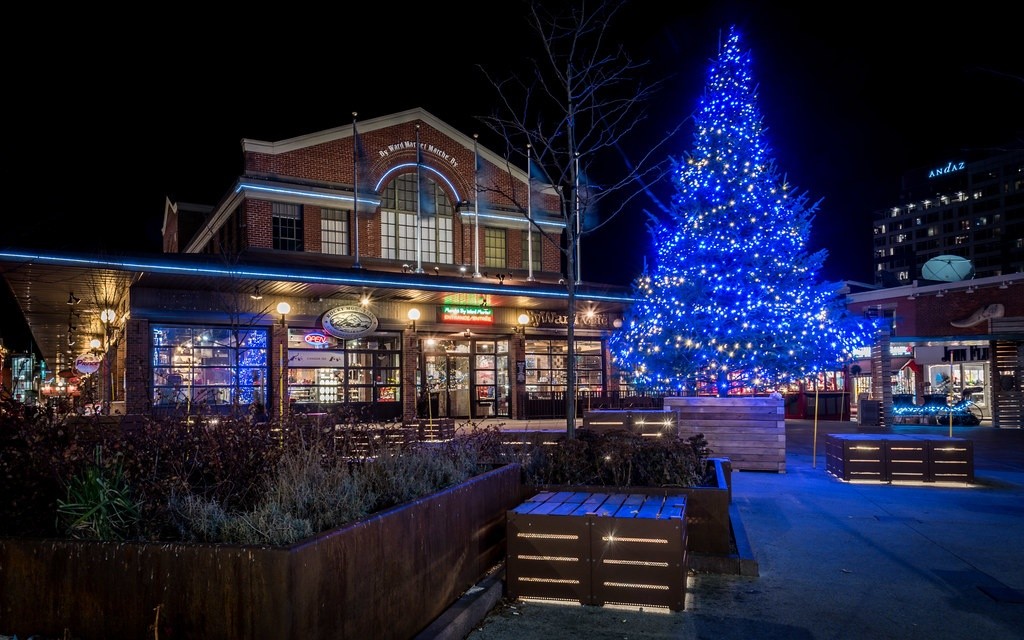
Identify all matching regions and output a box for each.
[275,301,291,329]
[89,338,104,357]
[99,306,119,339]
[517,313,530,337]
[408,308,422,331]
[614,318,624,337]
